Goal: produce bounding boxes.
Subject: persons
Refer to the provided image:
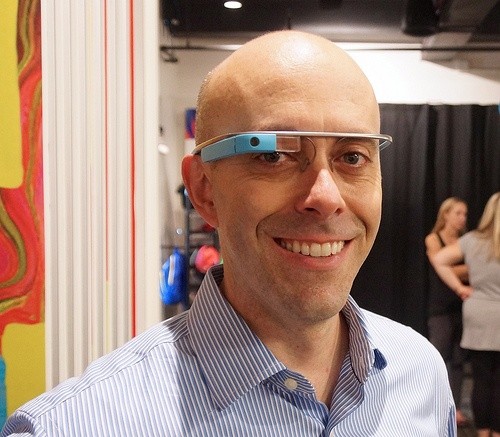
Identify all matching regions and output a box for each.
[423,199,470,424]
[1,29,457,436]
[435,192,500,436]
